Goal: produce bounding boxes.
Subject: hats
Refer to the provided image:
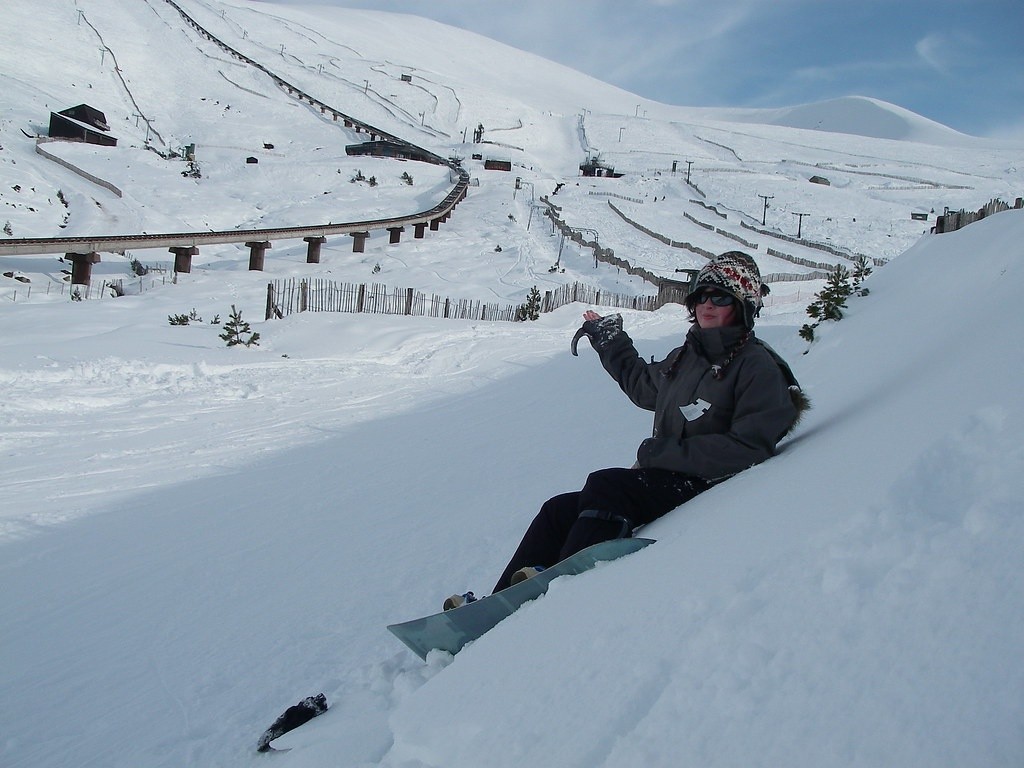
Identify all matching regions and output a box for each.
[688,251,767,326]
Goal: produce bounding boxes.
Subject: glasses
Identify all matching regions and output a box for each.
[693,289,739,307]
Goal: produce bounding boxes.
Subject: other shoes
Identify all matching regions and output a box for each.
[510,565,545,586]
[443,591,478,611]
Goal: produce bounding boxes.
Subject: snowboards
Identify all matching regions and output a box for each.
[381,531,663,669]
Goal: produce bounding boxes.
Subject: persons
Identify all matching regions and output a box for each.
[443,250,811,646]
